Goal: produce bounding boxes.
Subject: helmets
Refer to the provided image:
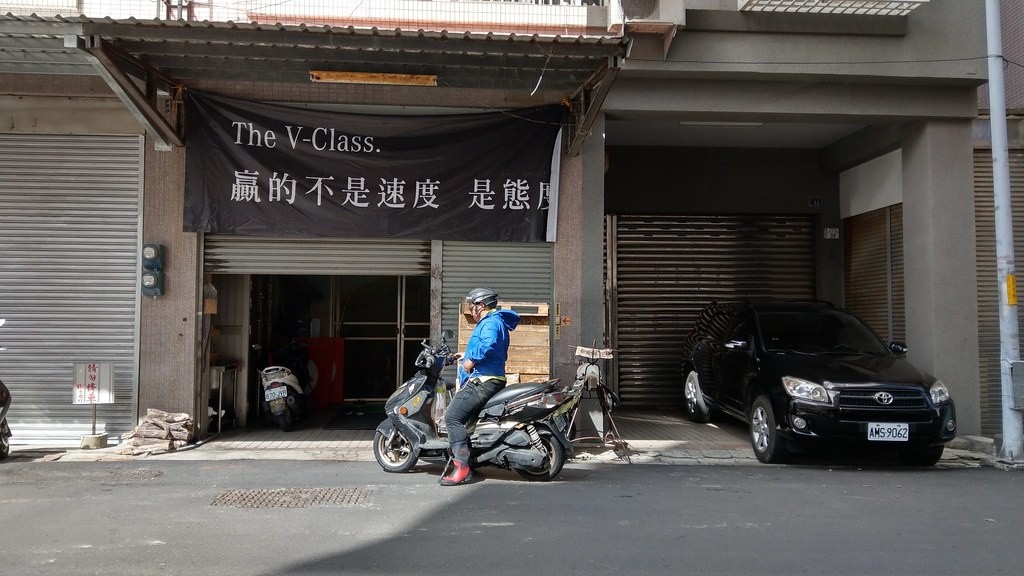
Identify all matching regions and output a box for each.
[465,288,498,309]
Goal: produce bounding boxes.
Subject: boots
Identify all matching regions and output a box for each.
[438,439,472,485]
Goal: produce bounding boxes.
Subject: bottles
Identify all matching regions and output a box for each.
[435,377,446,410]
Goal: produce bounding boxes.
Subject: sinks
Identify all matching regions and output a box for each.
[209,361,239,390]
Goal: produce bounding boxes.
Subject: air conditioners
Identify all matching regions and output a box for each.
[607,0,685,33]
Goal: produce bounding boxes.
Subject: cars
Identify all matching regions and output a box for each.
[679,294,958,471]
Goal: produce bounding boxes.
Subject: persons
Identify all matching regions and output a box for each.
[439,287,521,486]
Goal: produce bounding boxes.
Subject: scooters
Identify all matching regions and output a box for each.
[251,318,320,432]
[0,318,12,462]
[374,329,582,481]
